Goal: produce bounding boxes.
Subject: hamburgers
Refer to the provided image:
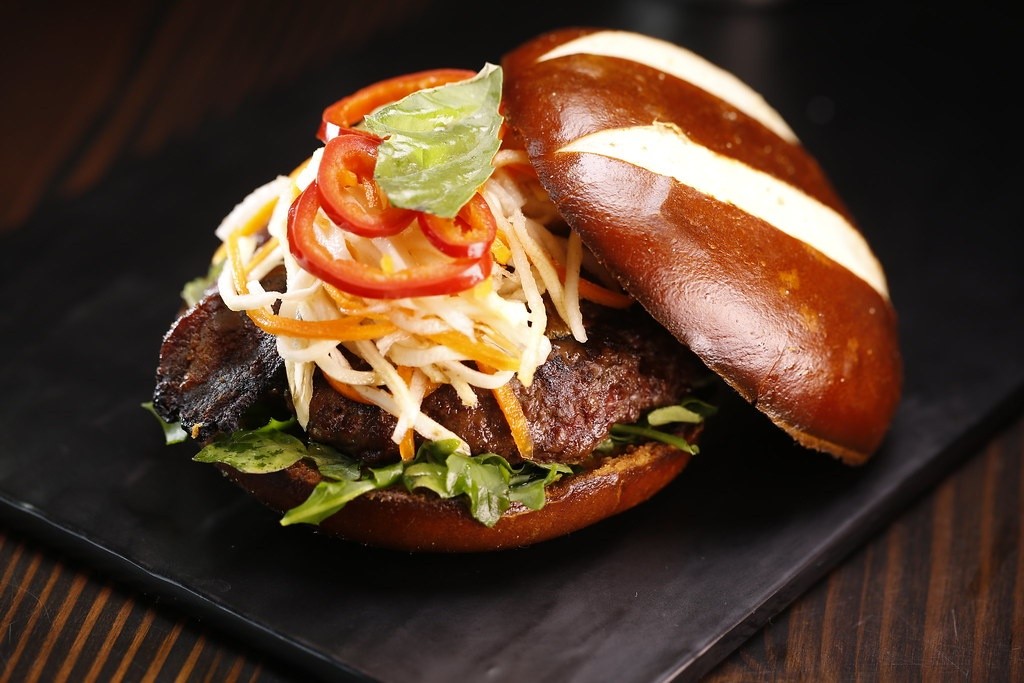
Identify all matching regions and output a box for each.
[142,30,902,553]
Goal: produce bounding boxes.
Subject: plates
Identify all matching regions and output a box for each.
[0,0,1024,683]
